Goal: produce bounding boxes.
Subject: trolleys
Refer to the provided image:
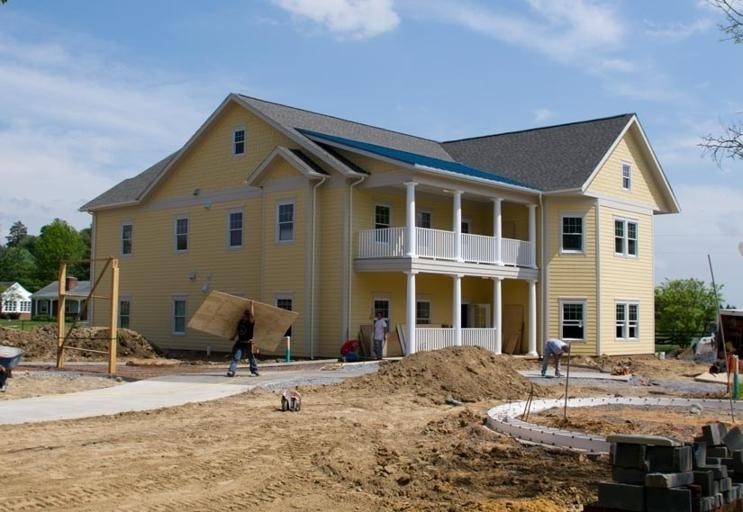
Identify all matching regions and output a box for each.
[0,344,24,389]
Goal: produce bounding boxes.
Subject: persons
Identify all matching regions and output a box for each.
[225,299,258,376]
[372,310,387,360]
[540,338,568,379]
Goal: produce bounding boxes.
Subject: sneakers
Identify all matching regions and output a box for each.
[227,371,258,377]
[542,371,564,376]
